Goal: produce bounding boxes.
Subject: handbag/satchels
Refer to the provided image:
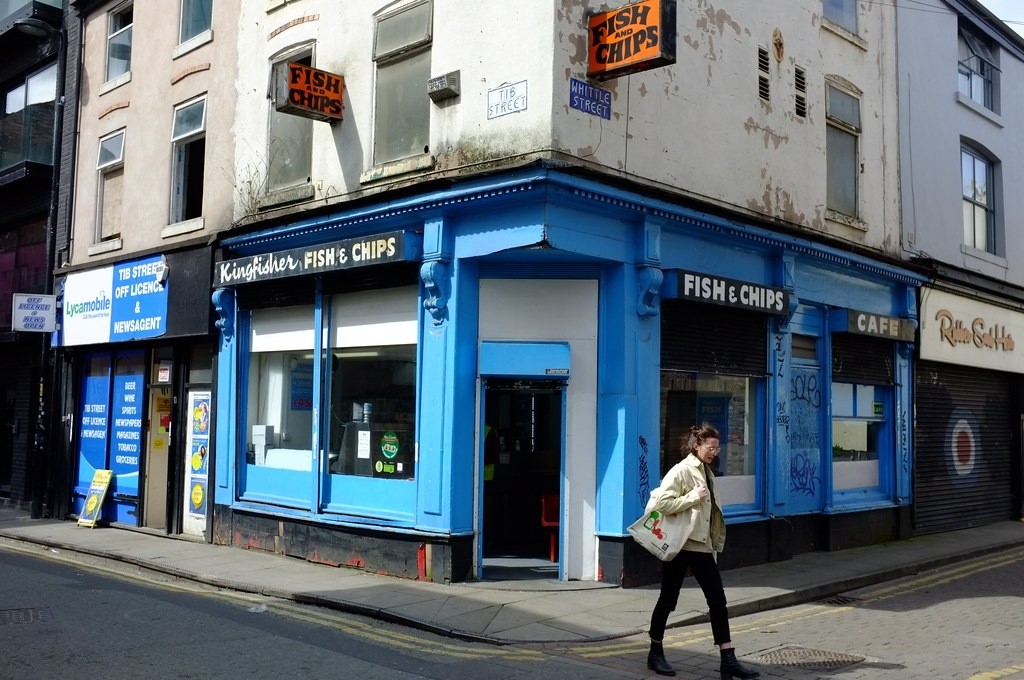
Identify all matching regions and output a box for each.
[626,467,701,561]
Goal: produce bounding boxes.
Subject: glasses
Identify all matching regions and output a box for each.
[700,444,720,453]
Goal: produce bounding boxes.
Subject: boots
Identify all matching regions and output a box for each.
[647,639,675,676]
[720,648,760,680]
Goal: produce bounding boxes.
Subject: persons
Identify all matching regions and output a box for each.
[373,406,412,478]
[484,424,497,480]
[648,425,761,680]
